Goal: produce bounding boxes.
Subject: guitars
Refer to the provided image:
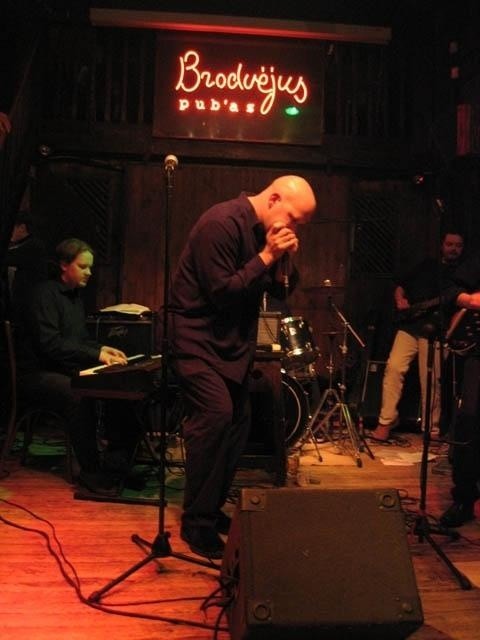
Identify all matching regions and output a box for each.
[390,291,449,325]
[442,290,480,356]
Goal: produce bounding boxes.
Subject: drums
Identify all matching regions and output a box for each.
[281,316,320,368]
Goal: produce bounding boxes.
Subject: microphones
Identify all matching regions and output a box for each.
[413,173,446,215]
[324,277,336,310]
[162,153,180,176]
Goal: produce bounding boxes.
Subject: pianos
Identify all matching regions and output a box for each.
[79,354,161,400]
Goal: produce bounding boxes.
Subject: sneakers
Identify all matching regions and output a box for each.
[214,510,233,535]
[180,522,226,560]
[439,502,474,527]
[368,418,400,441]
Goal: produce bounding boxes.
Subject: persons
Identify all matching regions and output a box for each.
[439,250,480,527]
[10,238,127,468]
[365,227,472,445]
[166,175,317,560]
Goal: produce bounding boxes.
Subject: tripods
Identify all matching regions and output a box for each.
[305,324,380,467]
[89,183,220,603]
[306,341,347,449]
[405,224,474,591]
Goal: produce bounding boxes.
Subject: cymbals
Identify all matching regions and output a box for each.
[306,285,341,295]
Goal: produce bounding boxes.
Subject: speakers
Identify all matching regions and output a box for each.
[215,486,425,640]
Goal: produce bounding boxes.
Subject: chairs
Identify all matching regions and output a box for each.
[3,321,73,482]
